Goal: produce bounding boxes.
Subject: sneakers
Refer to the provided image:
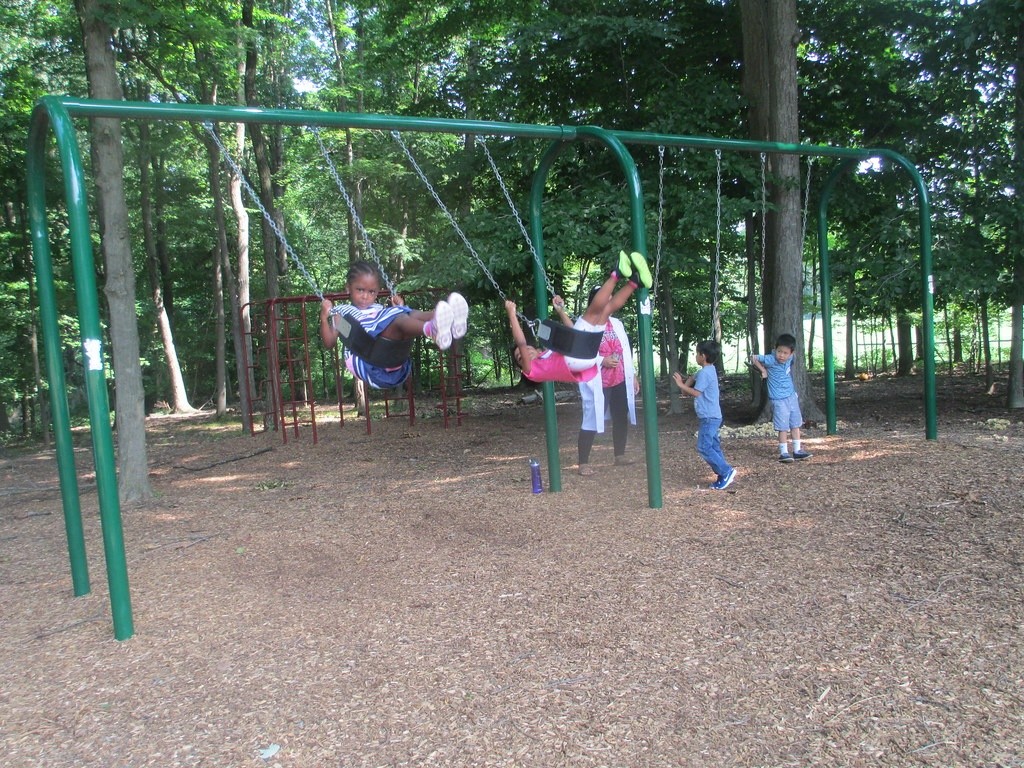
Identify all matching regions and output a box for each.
[708,469,737,490]
[431,292,468,350]
[628,252,653,289]
[778,453,794,463]
[615,250,632,279]
[793,450,813,460]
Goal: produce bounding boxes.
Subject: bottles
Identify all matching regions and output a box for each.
[529,458,542,493]
[612,346,622,360]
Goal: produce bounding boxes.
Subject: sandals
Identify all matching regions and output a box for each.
[578,464,594,476]
[615,455,635,464]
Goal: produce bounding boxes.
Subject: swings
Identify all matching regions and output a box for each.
[742,158,815,380]
[388,132,608,361]
[199,124,416,371]
[649,153,724,345]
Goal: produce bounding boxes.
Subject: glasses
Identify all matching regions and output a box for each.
[595,285,601,290]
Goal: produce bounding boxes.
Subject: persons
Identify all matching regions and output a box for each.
[319,260,469,390]
[752,333,814,463]
[504,250,653,384]
[672,340,737,490]
[574,284,640,476]
